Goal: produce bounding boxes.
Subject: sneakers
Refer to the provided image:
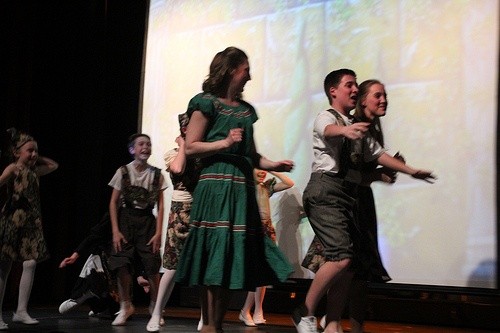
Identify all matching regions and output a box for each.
[320,315,326,329]
[290,310,318,333]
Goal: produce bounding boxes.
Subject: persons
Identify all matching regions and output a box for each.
[300,79,406,333]
[107,133,170,326]
[289,69,438,333]
[172,47,298,333]
[238,155,296,327]
[0,127,58,330]
[58,212,151,320]
[146,112,203,332]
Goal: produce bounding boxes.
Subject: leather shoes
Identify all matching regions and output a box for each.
[146,322,161,333]
[239,313,255,326]
[112,306,134,325]
[59,298,77,314]
[12,313,39,324]
[0,321,8,329]
[252,315,265,324]
